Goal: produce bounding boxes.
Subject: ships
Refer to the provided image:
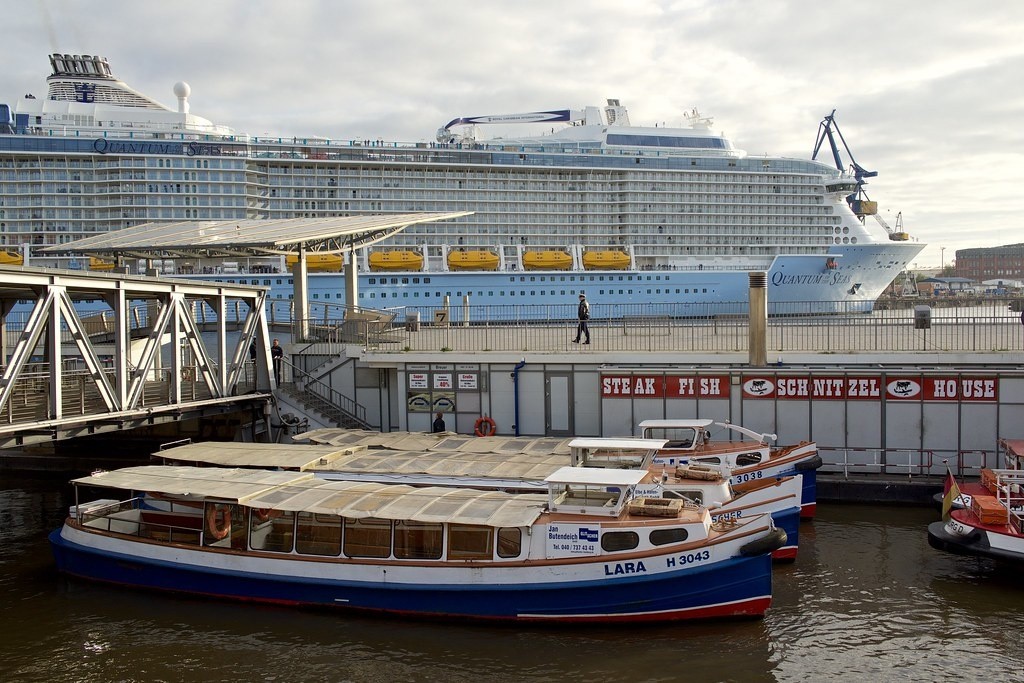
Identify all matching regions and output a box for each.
[0,48,930,339]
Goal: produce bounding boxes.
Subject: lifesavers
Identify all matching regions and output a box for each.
[473,417,496,436]
[258,509,273,520]
[207,503,230,539]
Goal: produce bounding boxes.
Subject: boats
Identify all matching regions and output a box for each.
[89,256,115,271]
[0,251,24,266]
[928,436,1024,571]
[369,250,423,272]
[583,251,630,271]
[522,250,573,271]
[44,416,824,624]
[447,250,500,271]
[286,252,343,272]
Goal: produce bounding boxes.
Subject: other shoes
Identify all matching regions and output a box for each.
[583,341,589,344]
[572,339,579,343]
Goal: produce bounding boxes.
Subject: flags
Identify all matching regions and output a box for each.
[942,467,961,521]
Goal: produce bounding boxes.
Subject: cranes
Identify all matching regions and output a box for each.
[861,199,909,241]
[811,108,878,224]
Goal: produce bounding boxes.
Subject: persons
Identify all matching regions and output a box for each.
[203,265,220,274]
[433,413,445,433]
[250,264,277,274]
[430,141,448,148]
[458,237,464,252]
[450,138,454,143]
[249,336,257,389]
[572,293,591,344]
[271,339,283,386]
[365,140,384,147]
[149,184,173,193]
[468,142,488,150]
